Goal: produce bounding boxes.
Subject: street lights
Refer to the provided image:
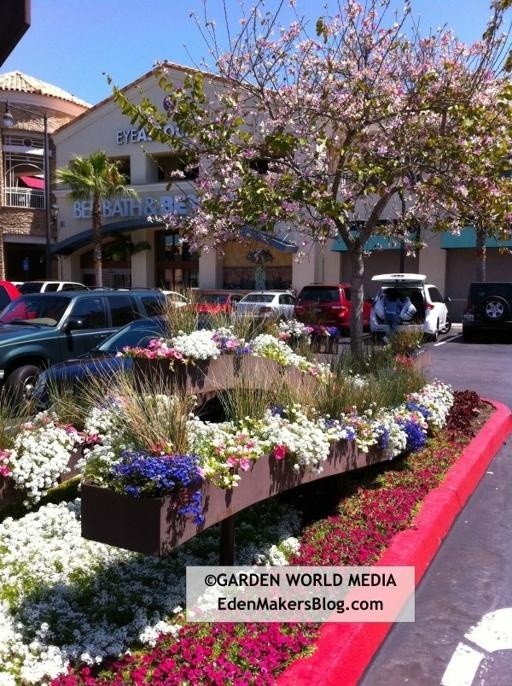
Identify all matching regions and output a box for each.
[2,99,52,280]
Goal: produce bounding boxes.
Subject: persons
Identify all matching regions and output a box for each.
[383,288,404,343]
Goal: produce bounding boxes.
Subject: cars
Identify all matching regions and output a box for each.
[293,273,452,345]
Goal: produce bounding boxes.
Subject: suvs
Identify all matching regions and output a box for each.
[463,282,512,343]
